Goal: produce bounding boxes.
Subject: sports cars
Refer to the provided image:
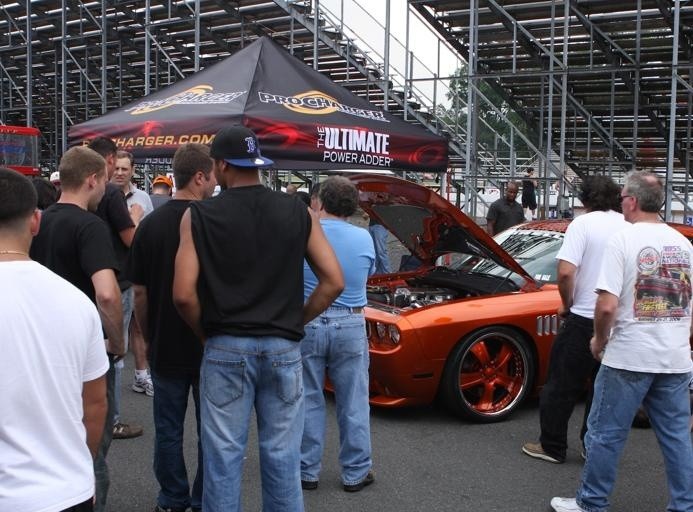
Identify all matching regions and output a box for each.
[320,171,693,421]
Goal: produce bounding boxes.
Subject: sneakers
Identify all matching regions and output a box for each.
[344,469,375,491]
[112,423,143,439]
[521,442,562,463]
[155,502,202,512]
[301,480,320,489]
[577,440,586,459]
[550,497,589,512]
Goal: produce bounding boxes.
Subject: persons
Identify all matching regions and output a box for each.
[174,123,344,512]
[86,136,144,440]
[367,216,395,275]
[29,146,126,512]
[148,173,174,211]
[521,174,634,464]
[0,167,107,512]
[129,143,228,512]
[551,172,693,512]
[28,170,62,210]
[485,167,574,236]
[107,151,155,396]
[295,177,384,493]
[283,180,319,214]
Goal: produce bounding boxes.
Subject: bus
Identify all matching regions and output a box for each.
[0,123,41,183]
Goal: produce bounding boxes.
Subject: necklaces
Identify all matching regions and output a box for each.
[1,249,30,256]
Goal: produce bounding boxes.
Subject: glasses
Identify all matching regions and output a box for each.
[617,193,632,202]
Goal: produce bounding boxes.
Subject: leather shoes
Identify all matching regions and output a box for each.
[132,369,154,396]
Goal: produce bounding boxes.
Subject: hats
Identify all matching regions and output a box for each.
[152,176,174,197]
[50,171,60,184]
[210,122,274,168]
